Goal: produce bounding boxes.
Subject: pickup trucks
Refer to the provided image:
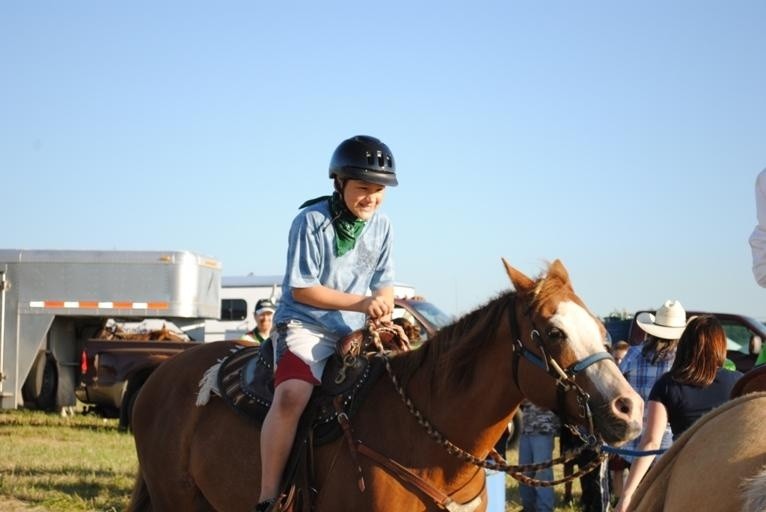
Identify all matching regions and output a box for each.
[74,296,463,434]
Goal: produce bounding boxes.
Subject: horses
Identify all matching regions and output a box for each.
[117,257,646,512]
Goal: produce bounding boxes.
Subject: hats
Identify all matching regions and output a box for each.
[636,299,688,340]
[255,299,276,317]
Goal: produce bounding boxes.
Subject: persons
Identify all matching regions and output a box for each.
[240,297,275,345]
[487,296,765,512]
[254,134,400,511]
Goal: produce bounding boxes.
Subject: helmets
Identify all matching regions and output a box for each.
[329,135,398,187]
